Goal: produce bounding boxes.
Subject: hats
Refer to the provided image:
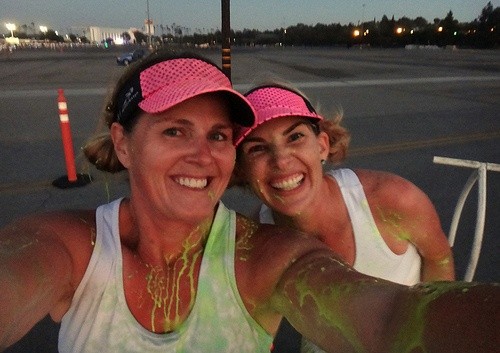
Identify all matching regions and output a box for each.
[113,54,257,128]
[234,88,322,147]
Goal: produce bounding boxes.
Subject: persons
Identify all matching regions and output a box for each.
[232,81,454,353]
[0,50,500,353]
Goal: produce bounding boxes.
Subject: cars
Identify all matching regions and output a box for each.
[117,47,152,66]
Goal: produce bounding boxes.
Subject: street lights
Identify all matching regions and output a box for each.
[6,23,19,42]
